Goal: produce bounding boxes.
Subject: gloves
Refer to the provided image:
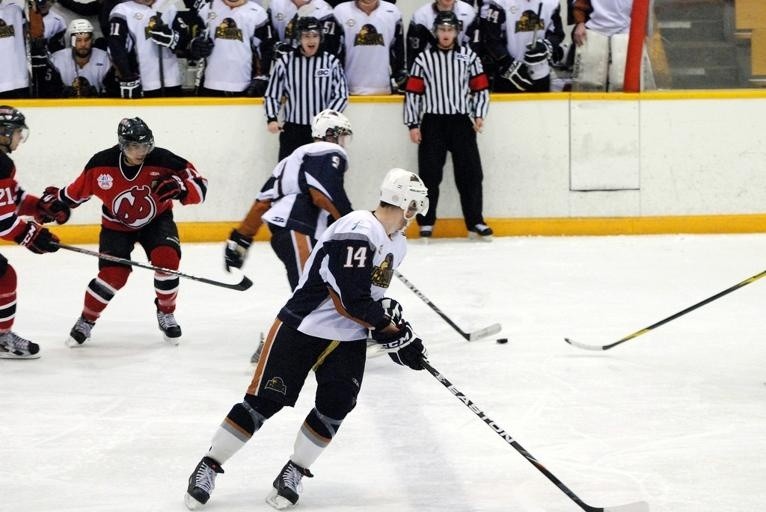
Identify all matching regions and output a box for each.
[377,298,417,338]
[151,23,181,50]
[35,184,72,225]
[188,35,215,62]
[381,320,433,371]
[149,171,191,204]
[17,219,61,258]
[119,80,143,99]
[222,227,254,272]
[524,37,554,67]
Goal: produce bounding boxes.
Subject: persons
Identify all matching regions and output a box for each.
[226,108,384,367]
[402,11,497,243]
[184,166,428,511]
[1,0,646,97]
[263,17,349,165]
[0,103,67,356]
[48,117,209,347]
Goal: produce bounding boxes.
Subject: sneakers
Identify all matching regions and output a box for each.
[187,458,222,505]
[0,334,41,357]
[467,222,496,236]
[68,317,93,346]
[272,457,311,506]
[157,312,185,342]
[420,225,434,238]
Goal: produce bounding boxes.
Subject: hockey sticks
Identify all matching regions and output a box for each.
[71,36,81,98]
[564,271,766,351]
[50,241,253,291]
[393,265,502,340]
[422,358,650,512]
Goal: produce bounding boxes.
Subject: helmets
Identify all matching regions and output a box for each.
[377,166,429,217]
[293,17,325,43]
[0,105,29,142]
[434,11,459,34]
[65,16,99,56]
[310,108,353,139]
[118,117,154,156]
